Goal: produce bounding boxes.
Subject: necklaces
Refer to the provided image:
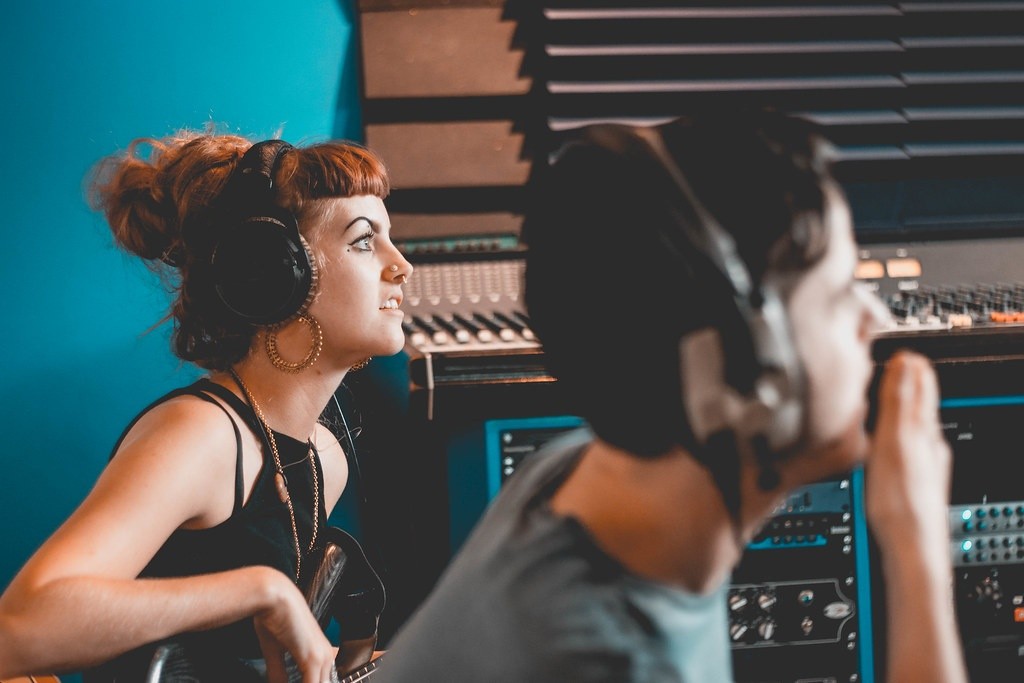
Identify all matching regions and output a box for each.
[227,365,319,586]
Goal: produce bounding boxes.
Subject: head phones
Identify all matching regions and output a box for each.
[207,139,320,329]
[586,122,809,495]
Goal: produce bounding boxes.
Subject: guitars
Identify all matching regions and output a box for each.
[147,541,393,681]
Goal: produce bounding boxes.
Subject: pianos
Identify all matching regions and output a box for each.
[403,252,876,378]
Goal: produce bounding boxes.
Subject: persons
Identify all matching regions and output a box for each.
[367,113,971,683]
[0,125,414,683]
